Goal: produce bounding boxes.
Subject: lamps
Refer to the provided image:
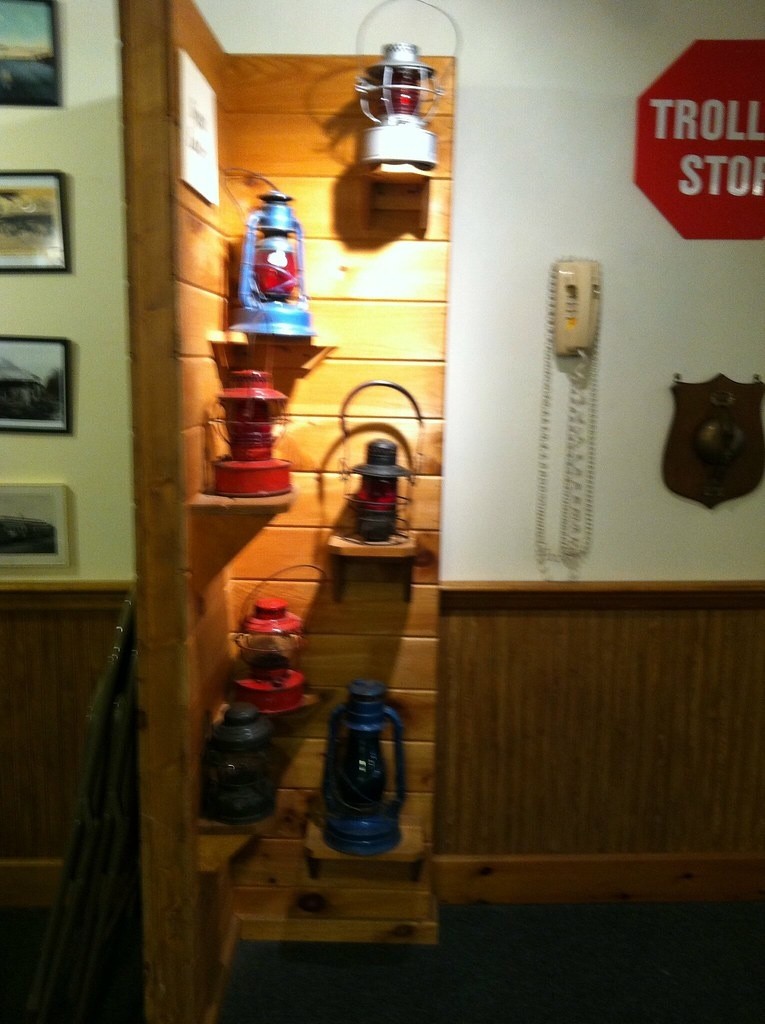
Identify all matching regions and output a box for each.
[338,380,427,548]
[226,563,327,714]
[222,164,319,339]
[320,677,407,855]
[204,705,278,824]
[351,0,461,168]
[208,369,291,496]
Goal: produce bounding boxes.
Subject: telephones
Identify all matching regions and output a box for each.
[556,260,603,358]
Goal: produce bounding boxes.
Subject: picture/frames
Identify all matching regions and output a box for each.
[0,336,70,433]
[0,484,70,569]
[0,169,70,273]
[0,0,61,108]
